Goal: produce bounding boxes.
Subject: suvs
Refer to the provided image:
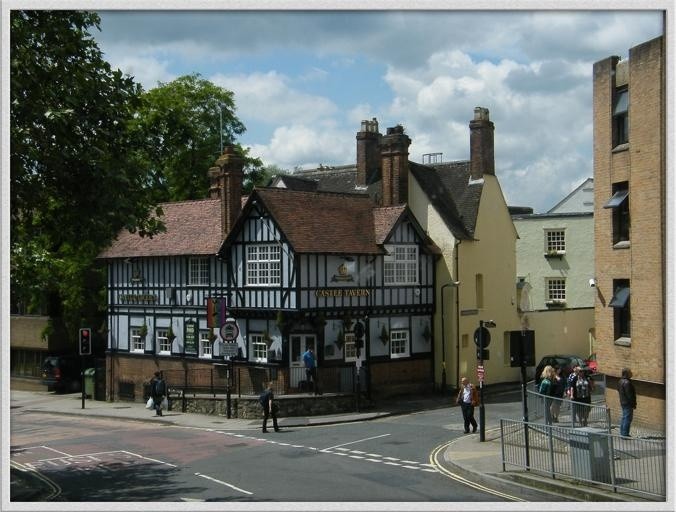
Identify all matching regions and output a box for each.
[37,352,97,393]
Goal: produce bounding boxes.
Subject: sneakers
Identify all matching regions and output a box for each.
[464,429,470,433]
[473,425,478,432]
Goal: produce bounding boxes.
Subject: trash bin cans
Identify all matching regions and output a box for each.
[568,426,612,486]
[84,367,95,399]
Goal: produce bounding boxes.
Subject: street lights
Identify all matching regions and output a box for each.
[475,319,496,442]
[441,280,461,397]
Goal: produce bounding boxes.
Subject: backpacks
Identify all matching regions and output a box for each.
[546,377,560,397]
[153,379,165,396]
[259,390,271,408]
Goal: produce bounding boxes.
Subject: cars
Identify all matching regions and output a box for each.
[535,352,587,387]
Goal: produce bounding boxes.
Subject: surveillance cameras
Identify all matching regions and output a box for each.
[588,278,595,287]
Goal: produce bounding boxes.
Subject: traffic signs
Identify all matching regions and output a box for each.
[218,342,238,357]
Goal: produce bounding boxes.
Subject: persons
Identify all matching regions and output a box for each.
[616,368,639,440]
[302,344,322,395]
[150,371,166,416]
[539,363,596,426]
[456,376,478,434]
[262,381,280,434]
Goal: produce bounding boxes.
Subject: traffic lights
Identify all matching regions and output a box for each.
[79,328,93,356]
